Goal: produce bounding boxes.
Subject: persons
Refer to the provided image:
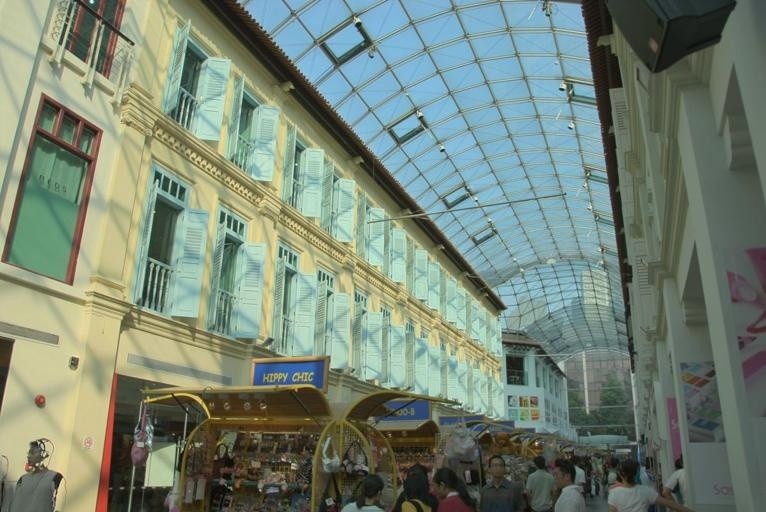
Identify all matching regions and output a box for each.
[607,460,695,512]
[526,456,558,512]
[662,453,689,512]
[10,440,64,512]
[554,459,587,512]
[340,474,386,512]
[392,464,477,512]
[483,473,493,489]
[480,456,528,512]
[555,453,663,497]
